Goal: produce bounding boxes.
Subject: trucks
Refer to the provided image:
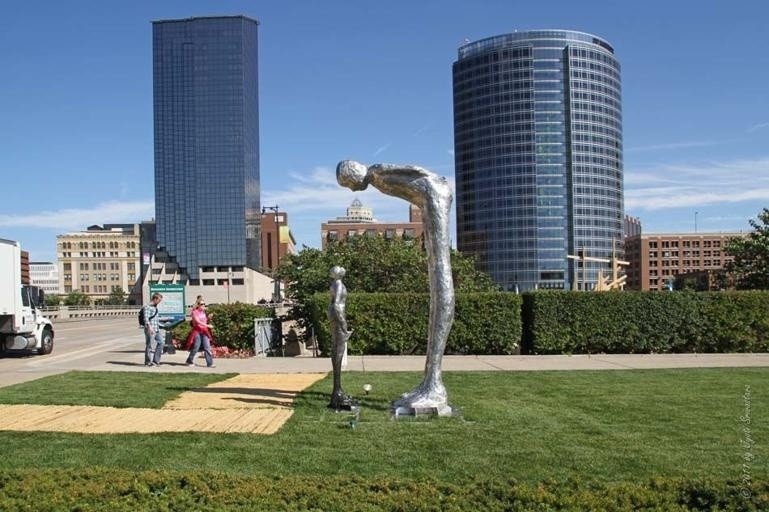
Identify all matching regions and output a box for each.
[0,236,56,356]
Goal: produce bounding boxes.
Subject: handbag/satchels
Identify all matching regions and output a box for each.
[138,307,158,326]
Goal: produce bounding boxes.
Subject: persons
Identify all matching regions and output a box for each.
[184,295,217,368]
[143,292,165,366]
[336,159,456,407]
[327,265,352,403]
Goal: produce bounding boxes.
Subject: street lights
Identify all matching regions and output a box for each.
[262,203,283,304]
